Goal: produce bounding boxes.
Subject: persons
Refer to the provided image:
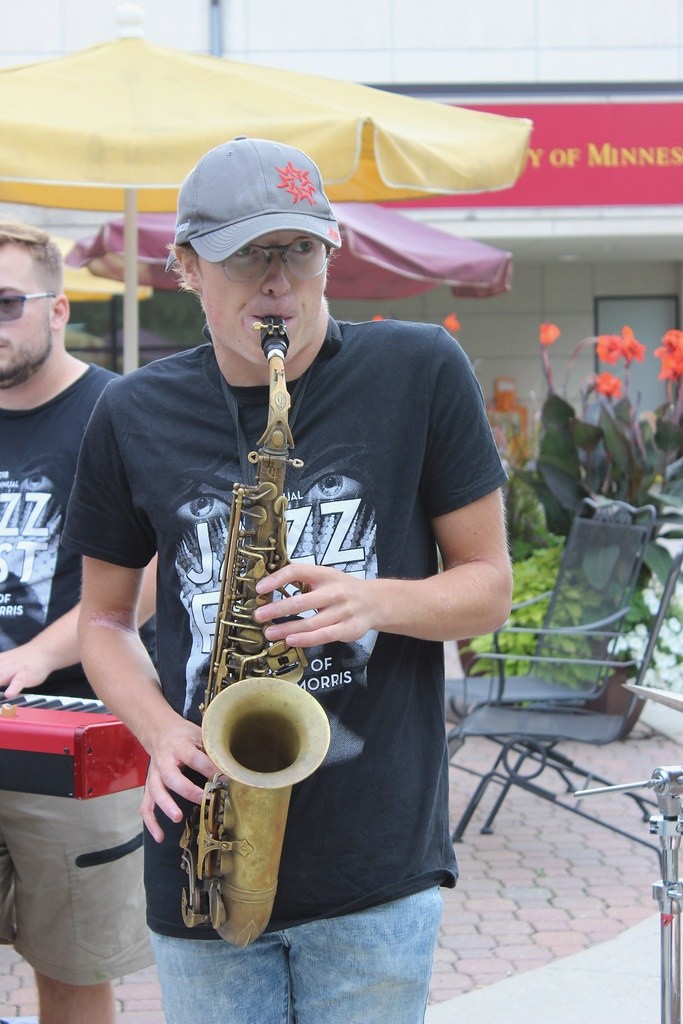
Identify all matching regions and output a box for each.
[0,221,159,1024]
[62,139,514,1023]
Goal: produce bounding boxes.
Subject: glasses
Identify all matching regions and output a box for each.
[0,291,57,322]
[222,236,329,284]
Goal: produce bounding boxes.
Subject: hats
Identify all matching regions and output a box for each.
[163,134,343,272]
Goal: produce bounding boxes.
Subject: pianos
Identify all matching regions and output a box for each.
[0,689,151,801]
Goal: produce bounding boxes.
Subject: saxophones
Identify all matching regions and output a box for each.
[176,314,334,952]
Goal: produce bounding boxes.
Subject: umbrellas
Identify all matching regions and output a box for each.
[1,37,534,379]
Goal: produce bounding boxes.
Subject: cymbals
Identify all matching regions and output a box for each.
[621,682,683,713]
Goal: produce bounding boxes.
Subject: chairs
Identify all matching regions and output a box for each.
[443,498,683,882]
